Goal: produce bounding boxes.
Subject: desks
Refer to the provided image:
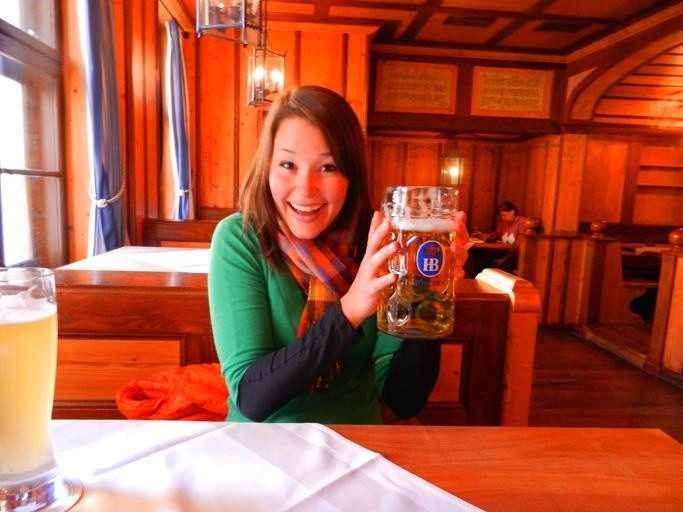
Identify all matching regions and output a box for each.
[0,420,683,512]
[51,205,541,426]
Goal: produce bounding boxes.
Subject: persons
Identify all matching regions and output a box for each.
[472,200,531,274]
[206,84,471,425]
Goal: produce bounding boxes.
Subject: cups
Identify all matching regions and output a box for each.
[1,264,89,511]
[375,182,459,340]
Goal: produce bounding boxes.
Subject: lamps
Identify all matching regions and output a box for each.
[246,0,287,110]
[192,0,266,47]
[441,125,469,187]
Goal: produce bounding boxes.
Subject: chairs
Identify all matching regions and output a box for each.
[494,216,546,264]
